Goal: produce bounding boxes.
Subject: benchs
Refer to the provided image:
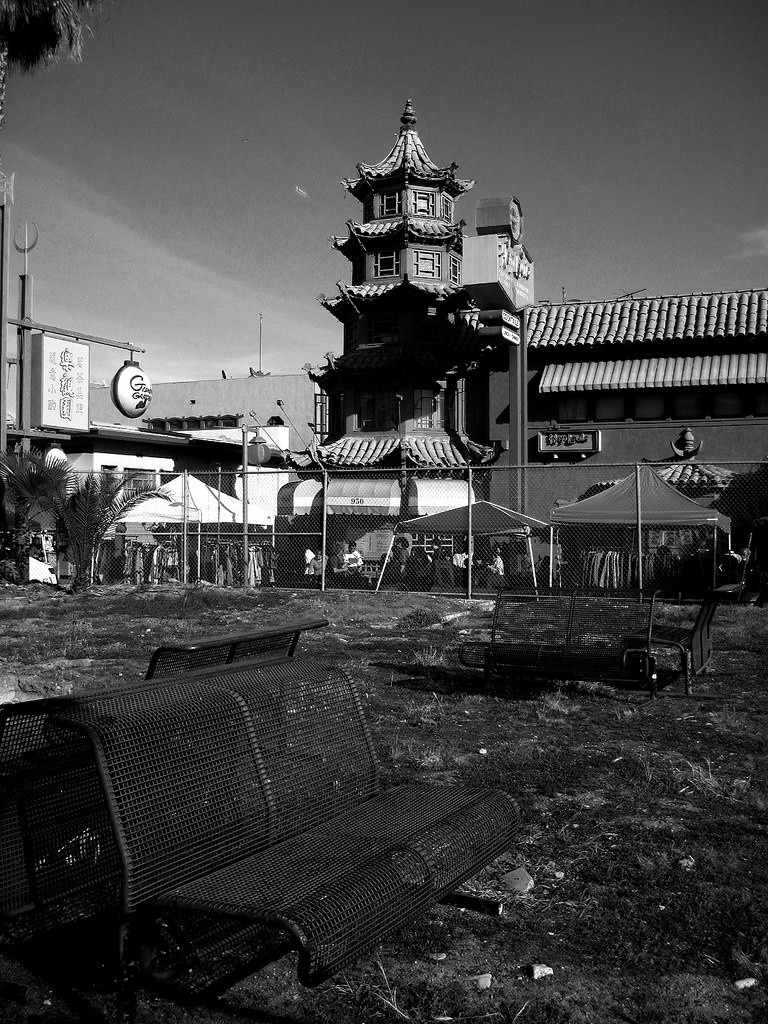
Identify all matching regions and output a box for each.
[0,656,295,948]
[620,590,733,695]
[458,587,661,700]
[145,619,329,681]
[43,659,522,1007]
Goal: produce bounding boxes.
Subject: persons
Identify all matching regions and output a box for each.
[304,536,506,596]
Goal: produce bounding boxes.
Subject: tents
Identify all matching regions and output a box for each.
[375,500,547,600]
[550,465,732,601]
[92,474,278,585]
